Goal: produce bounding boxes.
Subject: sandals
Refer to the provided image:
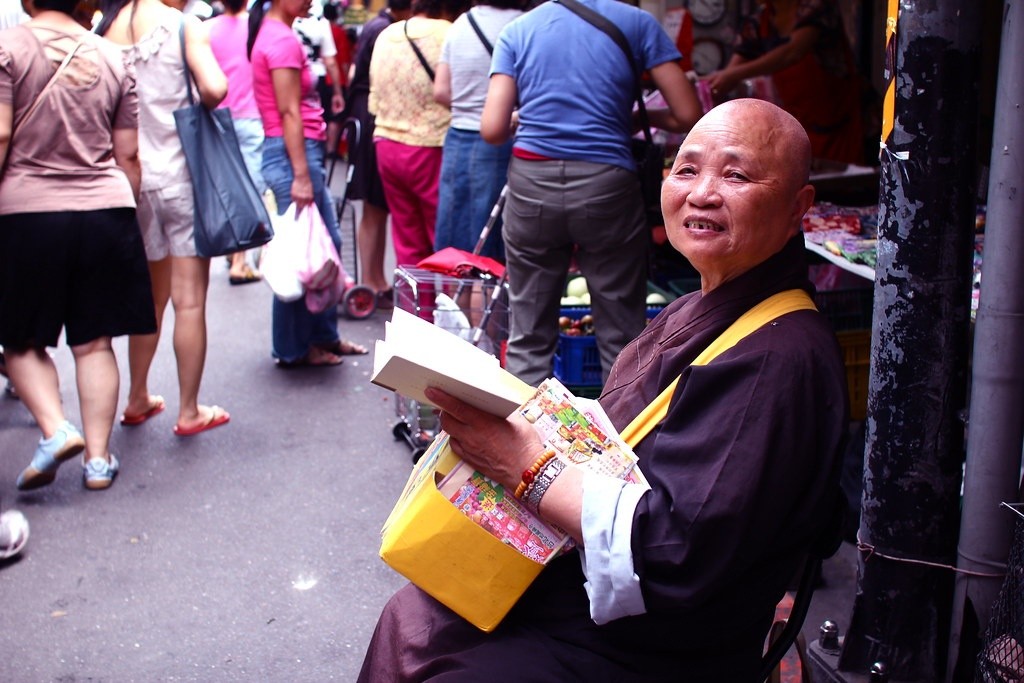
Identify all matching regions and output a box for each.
[326,338,369,355]
[276,345,345,368]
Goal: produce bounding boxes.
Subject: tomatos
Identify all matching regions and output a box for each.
[559,315,595,337]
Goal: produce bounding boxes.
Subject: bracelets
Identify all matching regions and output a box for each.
[514,450,568,515]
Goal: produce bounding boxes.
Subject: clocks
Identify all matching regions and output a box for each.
[690,35,725,77]
[685,0,728,29]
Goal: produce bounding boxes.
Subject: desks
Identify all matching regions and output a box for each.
[803,241,876,280]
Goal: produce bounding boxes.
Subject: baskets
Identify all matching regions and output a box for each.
[975,498,1024,682]
[553,303,664,387]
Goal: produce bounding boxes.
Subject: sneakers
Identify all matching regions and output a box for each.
[81,453,119,489]
[16,421,86,491]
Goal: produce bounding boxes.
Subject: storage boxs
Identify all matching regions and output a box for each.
[813,288,874,422]
[550,273,702,399]
[380,431,548,633]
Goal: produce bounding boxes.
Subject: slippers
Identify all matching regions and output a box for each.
[120,394,165,425]
[229,267,263,285]
[173,406,230,434]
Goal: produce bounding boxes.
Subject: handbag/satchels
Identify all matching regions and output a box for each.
[264,199,349,312]
[624,136,665,227]
[173,17,275,259]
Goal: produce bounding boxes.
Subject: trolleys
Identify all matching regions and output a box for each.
[390,177,510,465]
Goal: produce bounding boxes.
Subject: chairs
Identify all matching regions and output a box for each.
[748,486,849,683]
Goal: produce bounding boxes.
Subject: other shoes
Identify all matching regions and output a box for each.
[376,287,394,314]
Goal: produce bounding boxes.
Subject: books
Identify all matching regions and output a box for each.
[436,397,651,560]
[436,377,639,567]
[368,304,521,425]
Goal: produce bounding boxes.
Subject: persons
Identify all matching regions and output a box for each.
[701,0,865,174]
[355,98,851,683]
[200,1,370,367]
[0,0,230,490]
[348,0,704,389]
